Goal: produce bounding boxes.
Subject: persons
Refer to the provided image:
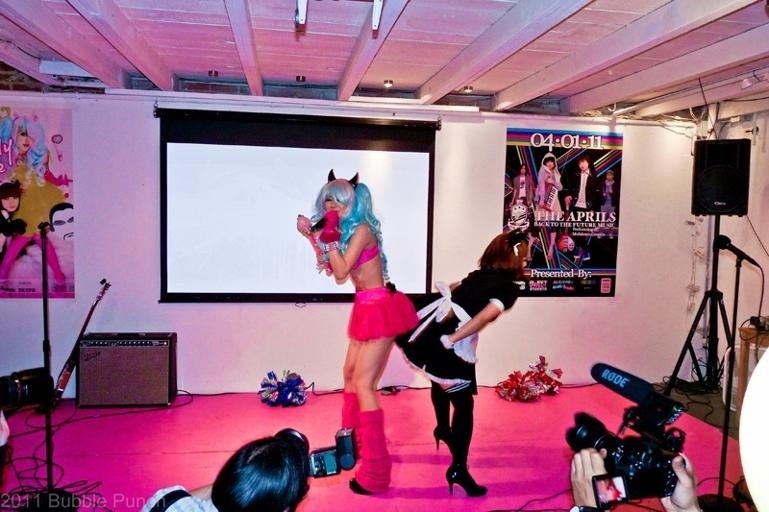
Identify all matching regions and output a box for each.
[296,167,409,492]
[400,231,555,498]
[511,155,619,283]
[0,104,74,300]
[136,436,311,511]
[568,446,706,512]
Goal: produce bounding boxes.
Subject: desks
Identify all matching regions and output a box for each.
[735,327,769,428]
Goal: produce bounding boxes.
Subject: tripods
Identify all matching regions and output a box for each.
[663,215,732,398]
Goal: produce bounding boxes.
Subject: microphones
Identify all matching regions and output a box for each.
[715,234,760,267]
[309,216,326,233]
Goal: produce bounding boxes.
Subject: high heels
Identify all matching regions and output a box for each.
[445,464,487,497]
[434,424,451,448]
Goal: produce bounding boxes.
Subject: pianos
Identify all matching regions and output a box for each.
[542,181,558,211]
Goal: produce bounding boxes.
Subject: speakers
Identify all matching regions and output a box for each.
[76,332,177,408]
[691,138,752,217]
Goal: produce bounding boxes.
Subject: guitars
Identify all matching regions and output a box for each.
[558,195,575,254]
[54,279,110,400]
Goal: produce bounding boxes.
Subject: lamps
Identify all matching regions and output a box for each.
[296,76,305,82]
[384,80,393,88]
[208,70,218,77]
[464,86,473,93]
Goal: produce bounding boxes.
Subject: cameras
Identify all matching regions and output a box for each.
[565,361,686,511]
[274,428,358,479]
